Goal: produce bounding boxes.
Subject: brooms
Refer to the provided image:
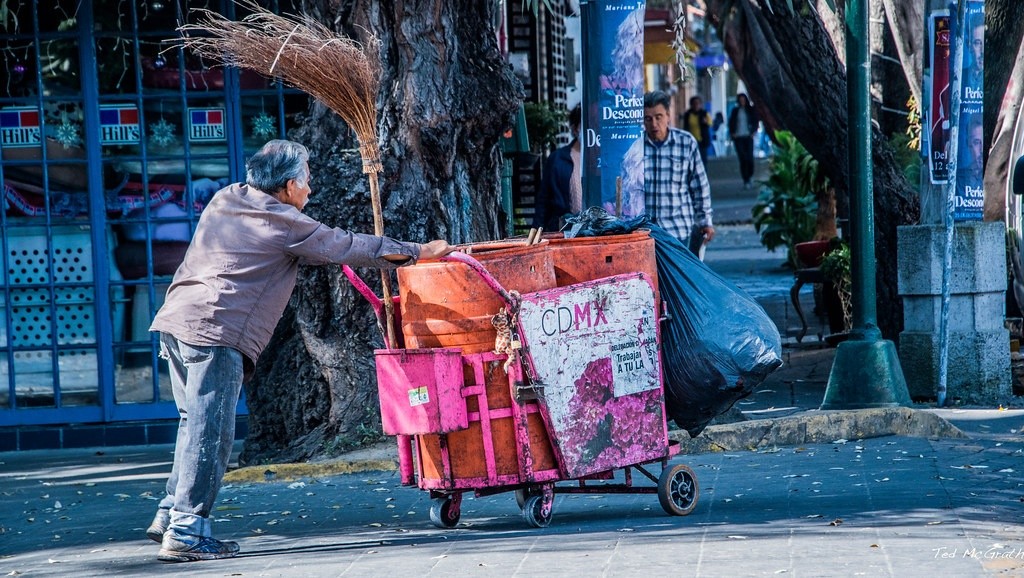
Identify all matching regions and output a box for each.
[158,1,395,349]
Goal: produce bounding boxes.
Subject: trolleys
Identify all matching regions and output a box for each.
[339,249,699,535]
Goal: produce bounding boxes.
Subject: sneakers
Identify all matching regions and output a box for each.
[158,529,240,561]
[146,510,170,543]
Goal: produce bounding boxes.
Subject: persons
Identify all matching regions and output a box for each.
[959,120,983,213]
[145,139,464,564]
[966,15,984,99]
[532,101,583,232]
[681,95,713,173]
[727,90,757,191]
[640,91,715,265]
